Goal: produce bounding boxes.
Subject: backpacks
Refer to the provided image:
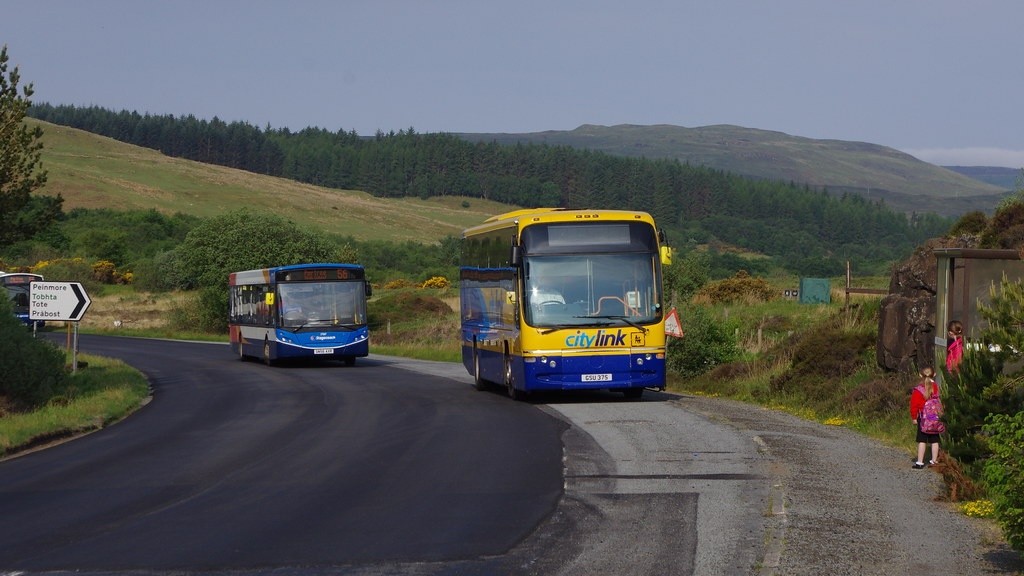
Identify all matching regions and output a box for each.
[913,384,945,434]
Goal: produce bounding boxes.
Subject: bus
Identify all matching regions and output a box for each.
[0,271,45,328]
[459,208,672,401]
[229,263,372,366]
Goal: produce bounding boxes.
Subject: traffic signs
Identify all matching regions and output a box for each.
[30,282,92,321]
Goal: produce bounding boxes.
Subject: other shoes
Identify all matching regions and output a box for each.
[911,462,925,469]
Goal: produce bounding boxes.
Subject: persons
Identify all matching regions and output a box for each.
[946,321,963,376]
[560,267,586,303]
[311,294,323,310]
[529,274,566,304]
[910,365,940,469]
[285,296,299,312]
